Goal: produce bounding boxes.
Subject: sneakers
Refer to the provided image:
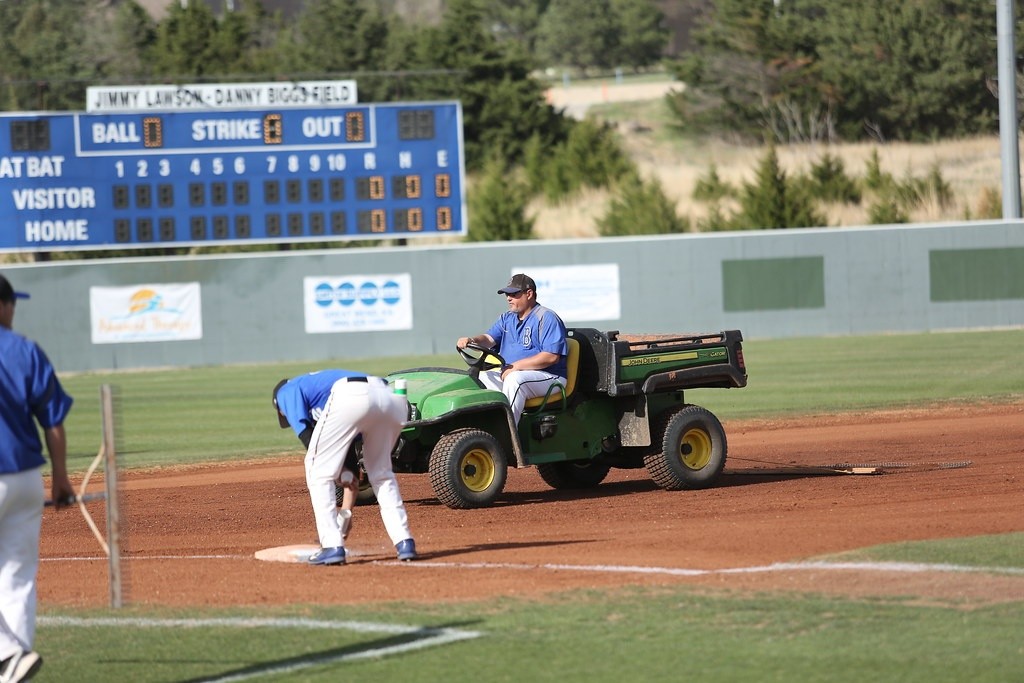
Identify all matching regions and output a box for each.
[0,650,43,683]
[395,539,416,561]
[308,545,346,566]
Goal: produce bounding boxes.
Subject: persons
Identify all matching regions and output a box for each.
[457,274,569,427]
[0,275,76,683]
[272,370,419,565]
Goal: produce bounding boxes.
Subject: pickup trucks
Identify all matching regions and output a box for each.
[334,327,748,512]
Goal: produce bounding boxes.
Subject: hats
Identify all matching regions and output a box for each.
[272,378,291,429]
[497,273,537,295]
[0,274,30,301]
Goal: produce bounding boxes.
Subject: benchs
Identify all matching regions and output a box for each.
[478,338,583,410]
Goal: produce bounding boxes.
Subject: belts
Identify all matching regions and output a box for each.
[348,377,388,383]
